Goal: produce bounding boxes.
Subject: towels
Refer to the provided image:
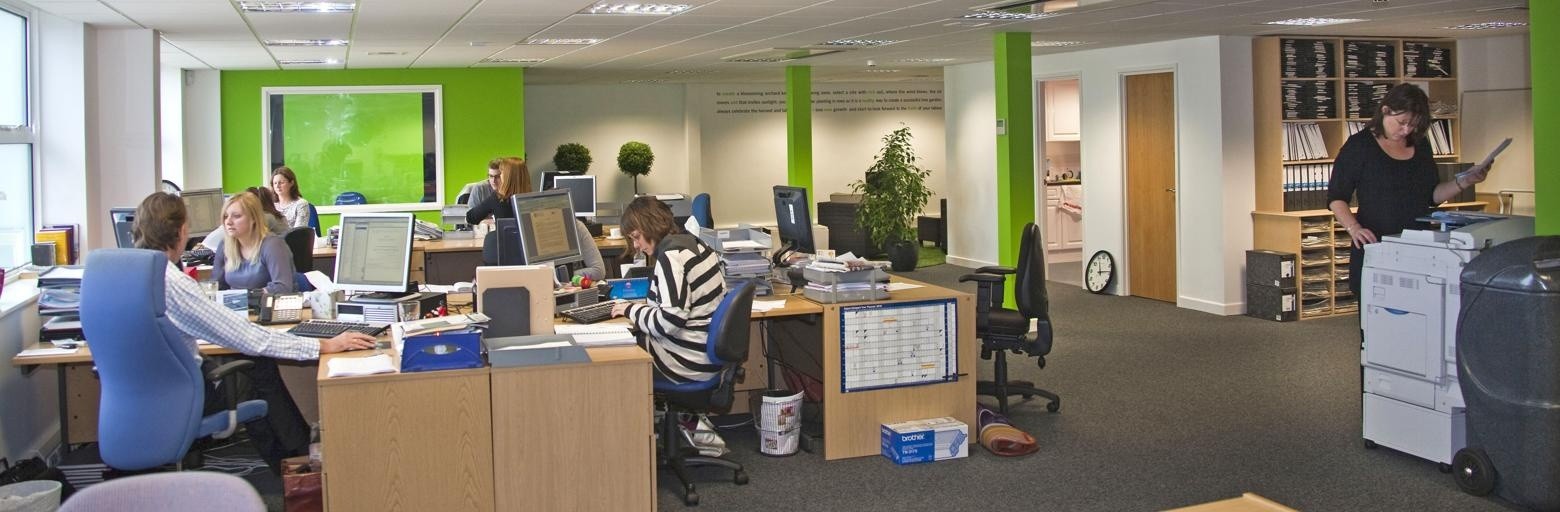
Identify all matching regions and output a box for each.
[1055,184,1084,218]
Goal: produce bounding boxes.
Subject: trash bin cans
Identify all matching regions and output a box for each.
[1454,235,1560,512]
[750,388,804,457]
[0,480,62,512]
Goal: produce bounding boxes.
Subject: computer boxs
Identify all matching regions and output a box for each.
[583,222,602,238]
[554,282,598,317]
[335,292,447,329]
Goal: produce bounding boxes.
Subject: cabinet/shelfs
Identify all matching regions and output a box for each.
[1045,182,1081,253]
[1246,199,1491,324]
[1250,34,1463,214]
[1043,78,1081,144]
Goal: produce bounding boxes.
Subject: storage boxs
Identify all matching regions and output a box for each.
[1244,284,1298,324]
[1245,246,1296,290]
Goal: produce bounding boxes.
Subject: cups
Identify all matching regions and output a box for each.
[1063,173,1067,180]
[397,299,421,323]
[610,227,622,238]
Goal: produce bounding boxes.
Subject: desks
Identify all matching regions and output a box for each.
[1160,490,1299,511]
[11,193,977,511]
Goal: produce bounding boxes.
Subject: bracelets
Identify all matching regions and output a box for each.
[1346,222,1359,232]
[1456,175,1471,191]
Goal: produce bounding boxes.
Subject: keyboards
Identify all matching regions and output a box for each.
[560,298,629,325]
[286,319,383,338]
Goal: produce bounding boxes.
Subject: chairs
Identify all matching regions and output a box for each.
[959,222,1062,421]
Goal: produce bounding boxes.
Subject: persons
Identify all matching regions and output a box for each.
[573,218,606,281]
[131,191,377,473]
[1326,82,1494,300]
[611,195,729,385]
[465,157,532,266]
[467,158,502,212]
[200,167,311,297]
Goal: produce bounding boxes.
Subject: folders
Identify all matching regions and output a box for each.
[1283,163,1334,211]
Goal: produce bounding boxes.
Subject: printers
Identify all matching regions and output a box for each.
[1357,213,1535,468]
[634,192,692,229]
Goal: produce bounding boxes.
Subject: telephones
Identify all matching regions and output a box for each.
[260,292,303,325]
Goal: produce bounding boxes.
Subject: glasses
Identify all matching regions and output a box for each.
[487,174,501,180]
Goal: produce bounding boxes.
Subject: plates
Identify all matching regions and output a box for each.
[607,236,626,240]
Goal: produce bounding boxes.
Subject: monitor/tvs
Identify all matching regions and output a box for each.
[554,175,597,217]
[224,194,232,202]
[497,218,526,265]
[110,208,136,248]
[510,188,584,286]
[177,188,223,238]
[540,171,580,190]
[773,185,816,267]
[333,212,418,300]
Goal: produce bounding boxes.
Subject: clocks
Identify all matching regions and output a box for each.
[1084,250,1116,296]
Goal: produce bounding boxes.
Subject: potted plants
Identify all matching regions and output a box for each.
[849,118,937,274]
